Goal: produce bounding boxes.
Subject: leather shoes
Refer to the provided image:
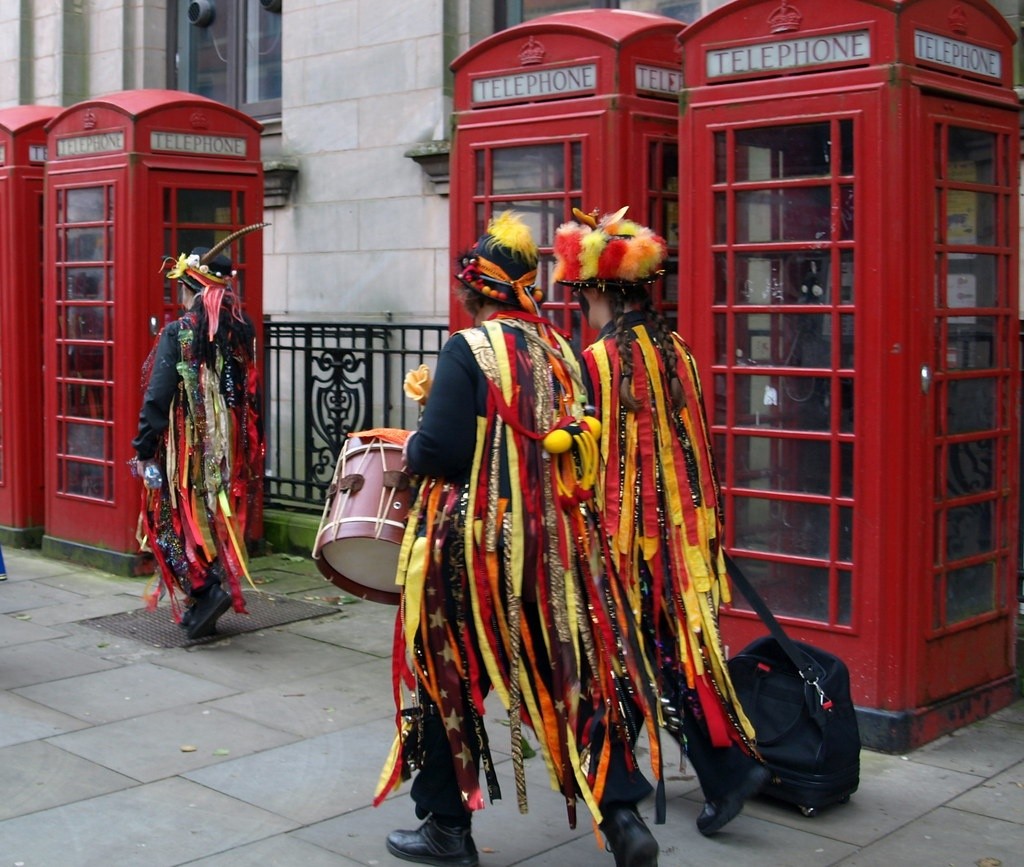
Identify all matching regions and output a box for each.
[385,814,479,865]
[598,805,659,865]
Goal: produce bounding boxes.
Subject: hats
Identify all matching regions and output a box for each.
[171,246,233,299]
[552,205,668,287]
[454,208,546,307]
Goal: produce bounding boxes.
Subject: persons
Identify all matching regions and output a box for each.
[386,234,659,867]
[131,247,267,639]
[569,233,775,836]
[67,273,114,427]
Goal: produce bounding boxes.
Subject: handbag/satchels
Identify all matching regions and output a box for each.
[723,634,861,814]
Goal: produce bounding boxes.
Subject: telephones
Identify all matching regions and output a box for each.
[785,256,859,344]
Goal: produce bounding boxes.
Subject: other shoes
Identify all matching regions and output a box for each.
[187,584,233,638]
[696,759,774,835]
[176,609,189,628]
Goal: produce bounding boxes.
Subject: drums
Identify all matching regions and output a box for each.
[310,427,427,607]
